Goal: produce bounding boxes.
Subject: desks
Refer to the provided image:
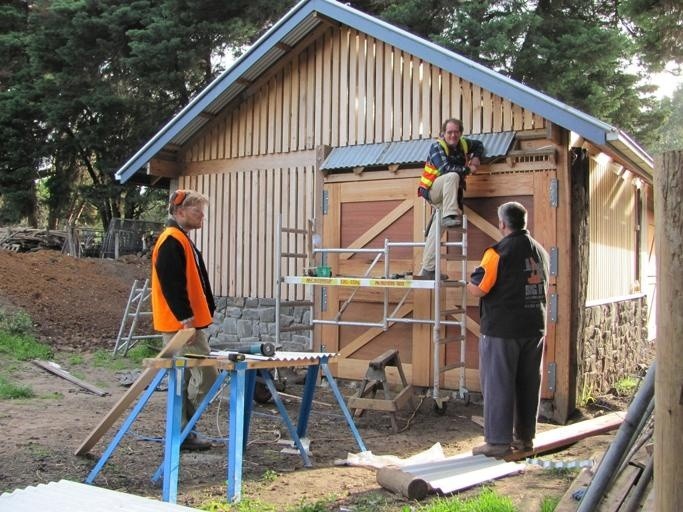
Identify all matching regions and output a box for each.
[86,357,367,508]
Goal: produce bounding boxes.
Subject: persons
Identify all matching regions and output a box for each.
[466,201,549,456]
[417,118,484,277]
[151,190,215,450]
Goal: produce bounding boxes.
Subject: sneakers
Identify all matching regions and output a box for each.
[422,269,449,280]
[442,217,461,228]
[472,444,513,456]
[512,435,533,451]
[180,433,212,450]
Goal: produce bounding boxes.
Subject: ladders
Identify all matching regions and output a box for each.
[113,278,163,357]
